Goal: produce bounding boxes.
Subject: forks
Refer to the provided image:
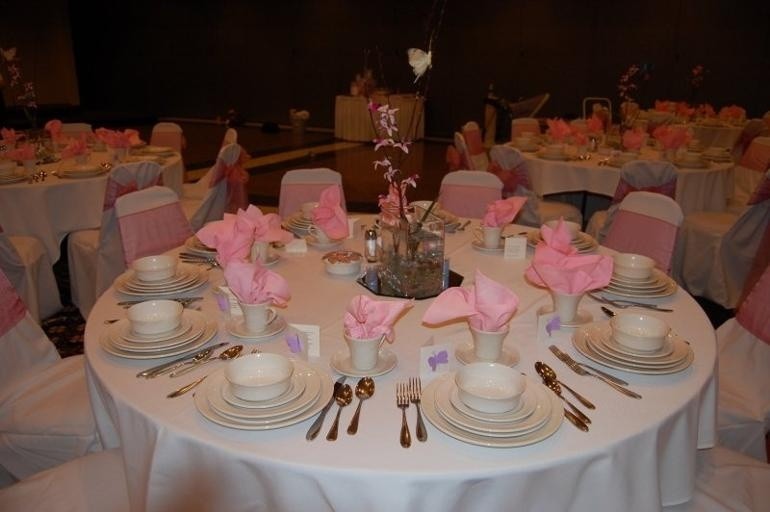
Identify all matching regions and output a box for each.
[394,377,427,448]
[547,344,644,402]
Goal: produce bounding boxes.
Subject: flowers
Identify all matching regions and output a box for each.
[0,46,38,128]
[347,31,443,288]
[611,61,642,135]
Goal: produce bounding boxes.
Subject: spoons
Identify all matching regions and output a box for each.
[327,374,375,441]
[533,361,598,432]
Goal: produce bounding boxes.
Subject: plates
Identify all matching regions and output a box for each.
[103,307,217,360]
[418,370,564,448]
[0,124,174,183]
[109,258,210,302]
[517,111,744,168]
[524,228,599,255]
[194,363,335,432]
[588,254,682,300]
[573,312,693,377]
[283,210,352,239]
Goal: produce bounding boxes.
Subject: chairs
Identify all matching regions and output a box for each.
[0,113,766,510]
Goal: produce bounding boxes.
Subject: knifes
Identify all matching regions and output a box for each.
[132,341,243,379]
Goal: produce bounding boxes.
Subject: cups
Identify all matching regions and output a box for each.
[307,224,333,246]
[471,223,503,247]
[235,296,276,331]
[250,239,271,263]
[549,289,581,323]
[347,329,389,367]
[469,319,510,362]
